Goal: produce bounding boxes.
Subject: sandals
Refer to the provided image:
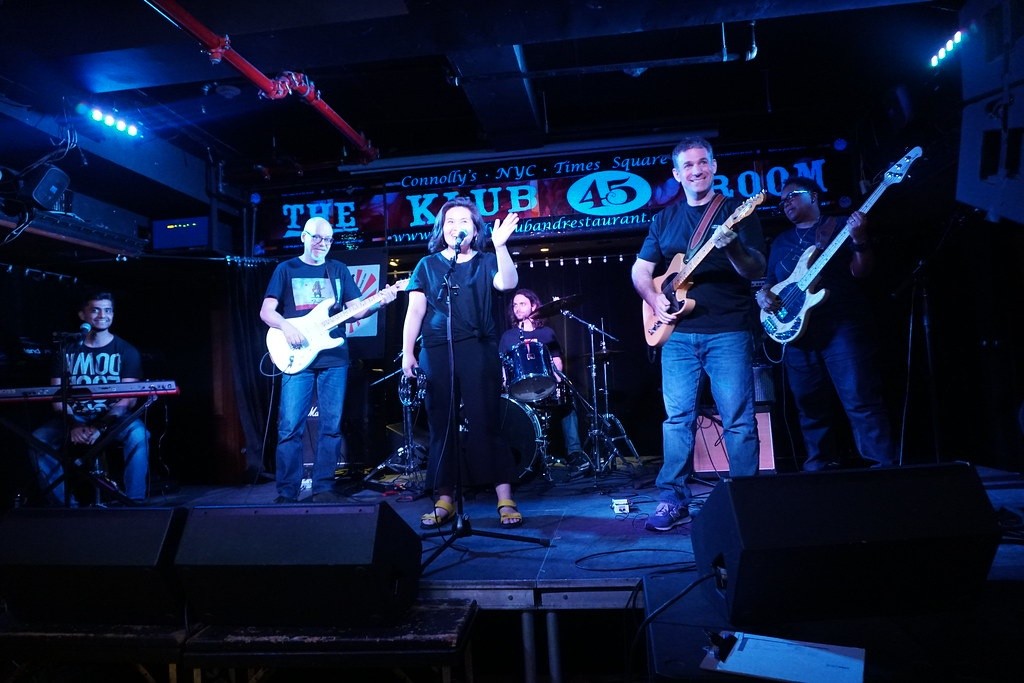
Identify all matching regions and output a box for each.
[497,499,522,528]
[421,500,456,528]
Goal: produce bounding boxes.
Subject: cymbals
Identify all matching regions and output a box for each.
[527,293,579,322]
[583,350,623,356]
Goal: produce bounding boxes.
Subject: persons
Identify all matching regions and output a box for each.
[498,289,590,468]
[631,137,765,530]
[259,217,398,504]
[401,199,523,530]
[755,176,894,472]
[27,291,150,504]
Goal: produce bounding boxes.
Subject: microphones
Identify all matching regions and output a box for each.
[77,323,91,348]
[519,322,524,339]
[456,231,467,245]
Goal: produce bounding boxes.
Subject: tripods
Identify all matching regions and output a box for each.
[363,368,428,483]
[419,244,550,580]
[556,305,644,484]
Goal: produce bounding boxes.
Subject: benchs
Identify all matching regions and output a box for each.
[0,620,204,683]
[186,602,476,683]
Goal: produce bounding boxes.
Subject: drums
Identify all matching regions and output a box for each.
[449,394,544,490]
[500,340,557,403]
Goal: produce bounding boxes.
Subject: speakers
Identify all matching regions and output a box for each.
[175,500,422,630]
[386,422,430,469]
[689,458,1004,626]
[300,401,349,469]
[16,161,70,211]
[0,504,189,634]
[956,0,1024,225]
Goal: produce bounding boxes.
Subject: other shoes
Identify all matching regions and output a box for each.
[567,456,590,476]
[311,493,343,504]
[272,495,299,504]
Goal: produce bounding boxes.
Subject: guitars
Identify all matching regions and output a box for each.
[642,188,769,346]
[266,279,411,375]
[760,146,923,343]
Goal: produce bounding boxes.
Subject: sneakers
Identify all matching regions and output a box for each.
[645,501,691,531]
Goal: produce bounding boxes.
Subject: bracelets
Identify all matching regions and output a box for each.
[755,288,767,299]
[853,240,871,252]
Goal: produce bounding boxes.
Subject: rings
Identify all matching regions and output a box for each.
[850,220,855,223]
[718,236,720,240]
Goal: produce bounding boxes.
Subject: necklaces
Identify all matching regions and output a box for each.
[796,222,815,243]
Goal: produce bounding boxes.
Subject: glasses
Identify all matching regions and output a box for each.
[305,231,334,245]
[778,190,814,209]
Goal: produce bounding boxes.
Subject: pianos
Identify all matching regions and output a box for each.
[0,377,181,508]
